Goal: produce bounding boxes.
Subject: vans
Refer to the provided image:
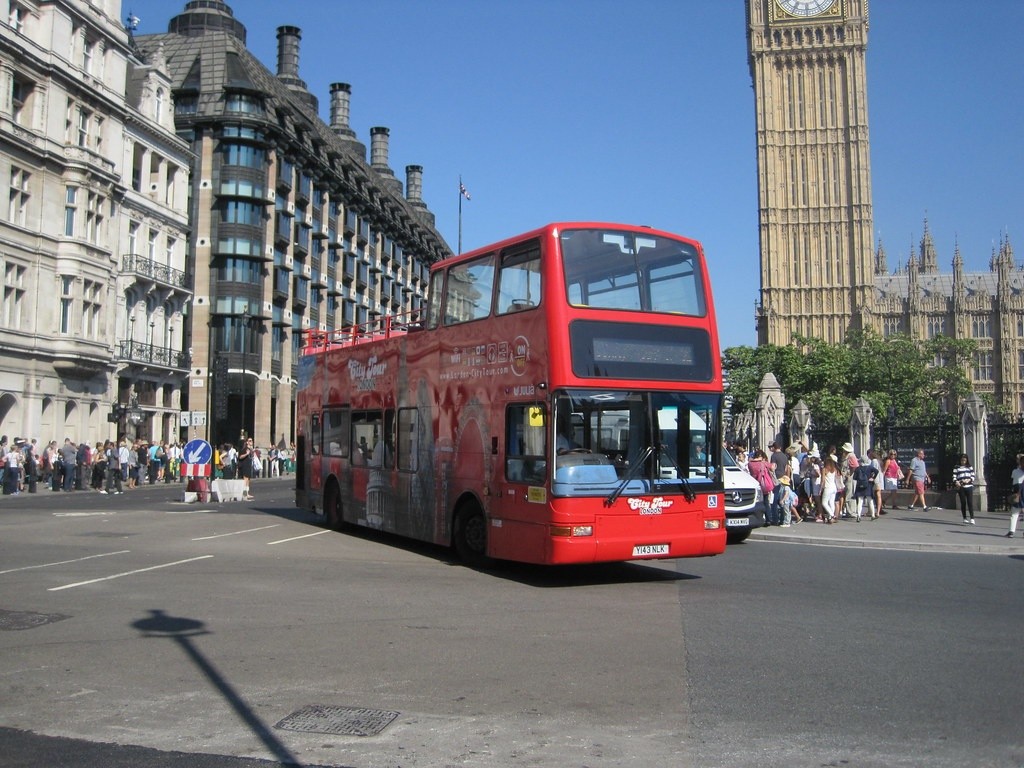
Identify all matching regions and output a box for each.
[570,405,766,546]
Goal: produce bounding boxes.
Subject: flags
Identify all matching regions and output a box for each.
[458,181,472,200]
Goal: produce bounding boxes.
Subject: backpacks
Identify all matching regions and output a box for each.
[783,486,799,507]
[857,466,868,490]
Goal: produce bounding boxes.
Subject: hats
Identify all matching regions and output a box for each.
[785,443,801,453]
[848,456,859,468]
[765,441,775,447]
[808,451,820,458]
[778,476,790,485]
[826,455,838,463]
[141,440,149,445]
[857,455,871,467]
[842,442,853,453]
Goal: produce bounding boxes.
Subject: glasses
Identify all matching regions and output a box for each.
[248,439,253,442]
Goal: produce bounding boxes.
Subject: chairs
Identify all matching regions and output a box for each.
[506,298,537,315]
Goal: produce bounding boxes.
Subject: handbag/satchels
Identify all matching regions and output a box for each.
[897,466,904,480]
[757,461,774,495]
[1007,493,1020,504]
[835,470,845,489]
[763,462,780,486]
[799,471,810,498]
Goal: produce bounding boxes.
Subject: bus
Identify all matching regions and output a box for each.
[295,220,728,566]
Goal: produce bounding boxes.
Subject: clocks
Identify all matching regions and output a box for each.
[776,0,838,17]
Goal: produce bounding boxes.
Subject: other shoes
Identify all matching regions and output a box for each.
[963,519,975,525]
[1007,532,1013,537]
[892,506,899,509]
[923,507,932,512]
[908,505,914,510]
[8,484,138,495]
[765,510,888,527]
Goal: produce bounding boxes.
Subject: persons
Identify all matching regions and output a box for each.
[693,442,706,461]
[906,449,932,512]
[556,422,569,455]
[722,440,888,529]
[99,442,124,495]
[883,449,901,510]
[268,442,295,477]
[1005,453,1024,538]
[351,436,368,465]
[0,435,264,502]
[952,454,977,524]
